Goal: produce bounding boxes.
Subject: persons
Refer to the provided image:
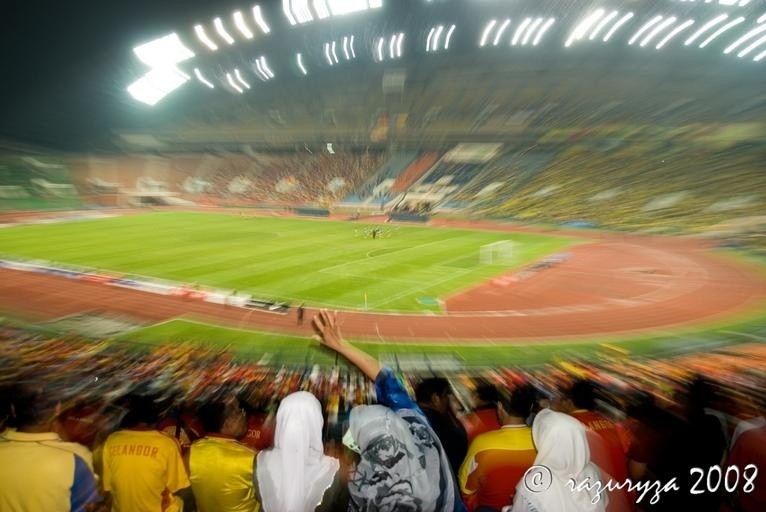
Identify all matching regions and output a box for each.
[1,57,765,511]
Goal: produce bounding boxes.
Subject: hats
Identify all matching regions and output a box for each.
[499,386,536,416]
[564,381,599,410]
